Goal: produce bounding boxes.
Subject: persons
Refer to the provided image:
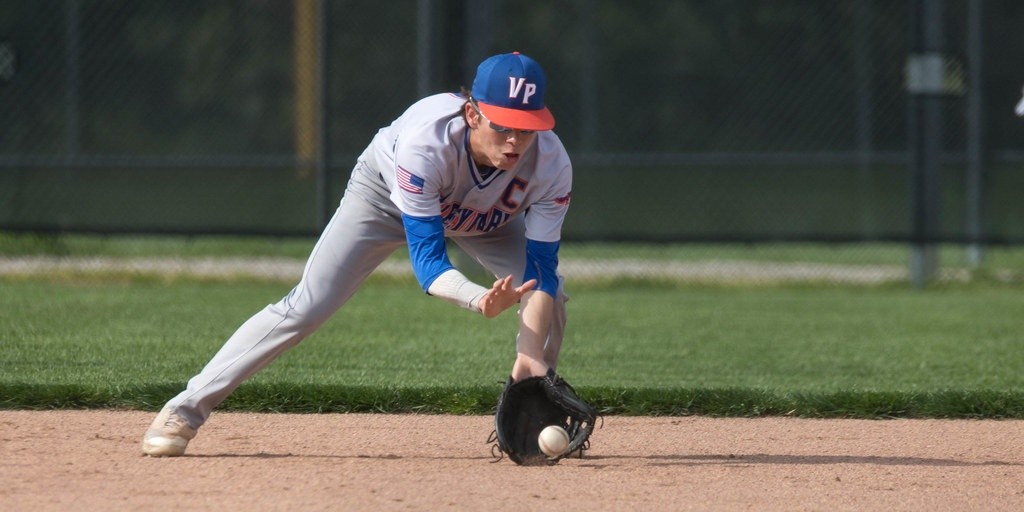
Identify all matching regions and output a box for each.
[141,53,573,457]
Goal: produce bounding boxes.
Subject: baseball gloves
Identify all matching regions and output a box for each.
[486,369,606,466]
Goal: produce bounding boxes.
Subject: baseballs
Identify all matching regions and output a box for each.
[538,425,569,455]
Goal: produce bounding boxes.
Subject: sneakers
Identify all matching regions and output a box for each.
[142,405,197,458]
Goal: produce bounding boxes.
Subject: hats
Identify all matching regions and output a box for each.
[471,52,555,131]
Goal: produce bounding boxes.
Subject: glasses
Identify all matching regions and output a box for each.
[470,97,535,135]
